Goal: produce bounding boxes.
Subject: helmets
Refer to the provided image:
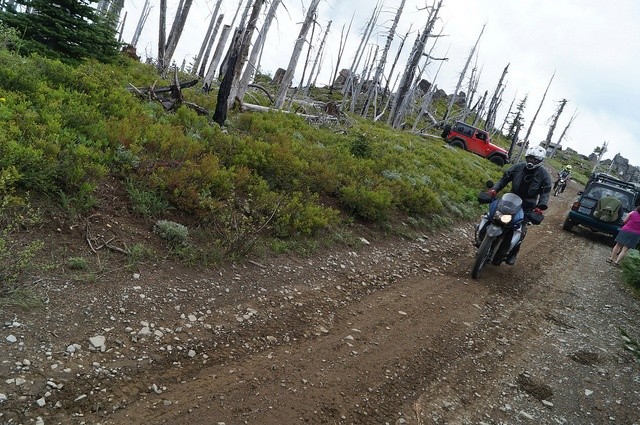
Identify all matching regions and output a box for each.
[566,165,572,172]
[525,146,546,169]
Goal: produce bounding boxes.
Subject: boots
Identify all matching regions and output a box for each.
[506,240,522,264]
[473,237,481,246]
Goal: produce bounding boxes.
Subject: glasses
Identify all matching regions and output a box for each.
[526,155,541,163]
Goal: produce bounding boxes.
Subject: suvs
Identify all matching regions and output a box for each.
[441,121,511,167]
[563,181,638,246]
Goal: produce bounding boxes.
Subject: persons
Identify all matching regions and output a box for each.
[472,144,552,265]
[553,164,573,196]
[606,206,640,267]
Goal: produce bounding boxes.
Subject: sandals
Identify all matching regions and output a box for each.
[606,259,612,263]
[613,263,621,268]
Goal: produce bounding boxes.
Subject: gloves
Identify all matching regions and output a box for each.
[535,208,542,215]
[488,188,496,197]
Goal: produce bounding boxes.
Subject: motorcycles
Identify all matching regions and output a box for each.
[470,180,548,280]
[553,174,572,197]
